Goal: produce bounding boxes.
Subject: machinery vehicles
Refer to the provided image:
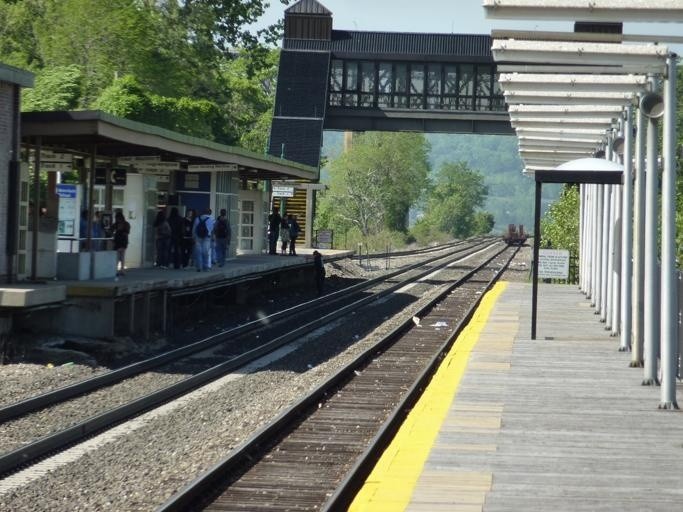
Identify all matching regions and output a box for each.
[498,223,530,247]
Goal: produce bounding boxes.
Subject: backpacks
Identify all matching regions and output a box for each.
[216,219,228,238]
[196,216,209,238]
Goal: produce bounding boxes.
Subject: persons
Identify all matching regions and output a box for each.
[279,212,288,255]
[288,215,300,256]
[267,208,278,255]
[80,209,109,239]
[149,205,230,272]
[109,213,129,276]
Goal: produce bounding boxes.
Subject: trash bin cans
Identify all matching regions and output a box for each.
[91,236,118,280]
[57,235,91,282]
